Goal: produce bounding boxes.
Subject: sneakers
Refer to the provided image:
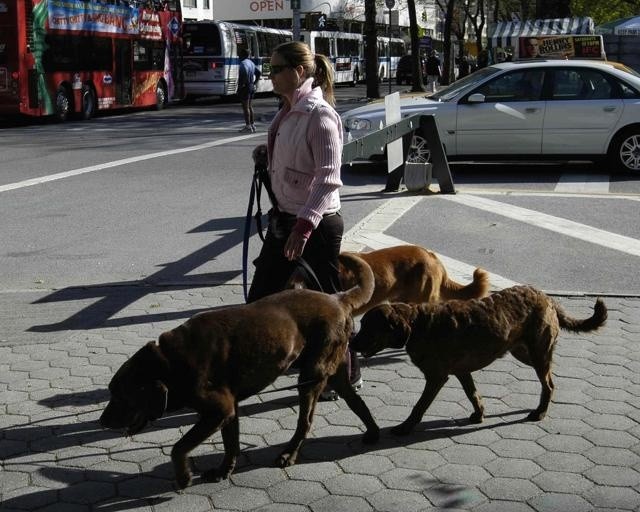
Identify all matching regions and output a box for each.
[319,372,363,402]
[240,126,256,134]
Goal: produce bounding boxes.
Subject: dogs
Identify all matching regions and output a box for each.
[97,251,381,494]
[351,284,609,436]
[286,244,486,318]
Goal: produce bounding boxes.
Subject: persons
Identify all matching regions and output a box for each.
[477,45,490,69]
[247,42,364,401]
[522,37,544,59]
[423,49,442,92]
[237,47,261,133]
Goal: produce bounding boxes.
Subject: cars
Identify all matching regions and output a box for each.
[339,58,640,177]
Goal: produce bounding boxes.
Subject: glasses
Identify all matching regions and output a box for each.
[268,64,290,75]
